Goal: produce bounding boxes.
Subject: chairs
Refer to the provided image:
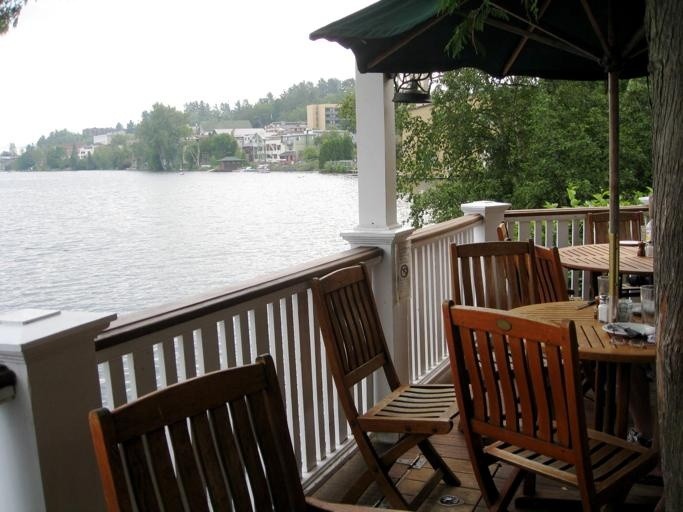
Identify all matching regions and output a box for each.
[588,211,645,296]
[89,353,408,512]
[497,220,510,241]
[442,300,662,511]
[449,242,582,431]
[311,261,489,512]
[534,243,570,303]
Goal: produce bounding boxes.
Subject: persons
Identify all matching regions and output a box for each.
[626,363,653,448]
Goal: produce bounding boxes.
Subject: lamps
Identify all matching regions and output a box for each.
[392,72,432,104]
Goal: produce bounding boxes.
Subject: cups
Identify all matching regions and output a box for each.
[639,285,657,327]
[597,276,609,296]
[641,226,651,243]
[616,303,632,322]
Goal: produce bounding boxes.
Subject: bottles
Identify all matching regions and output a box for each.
[598,296,608,322]
[637,240,646,256]
[594,296,600,319]
[645,241,653,258]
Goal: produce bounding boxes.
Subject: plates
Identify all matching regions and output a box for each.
[619,240,640,245]
[618,302,655,314]
[601,322,655,337]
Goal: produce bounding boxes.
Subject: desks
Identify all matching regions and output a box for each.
[558,242,653,299]
[506,299,656,441]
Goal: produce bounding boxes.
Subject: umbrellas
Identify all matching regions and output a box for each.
[310,0,650,324]
